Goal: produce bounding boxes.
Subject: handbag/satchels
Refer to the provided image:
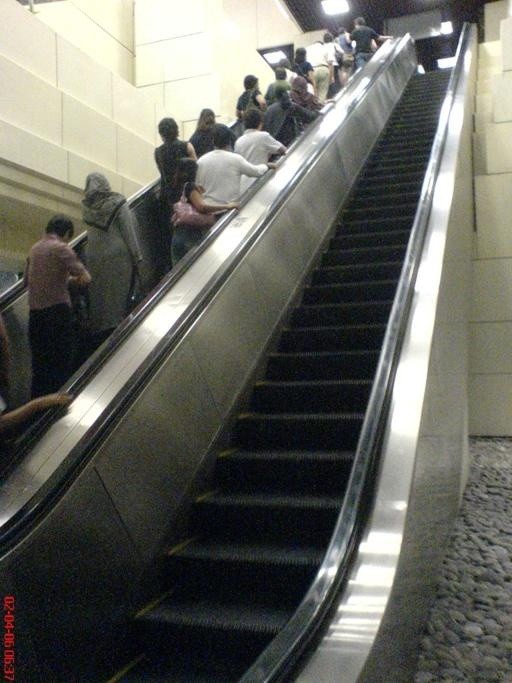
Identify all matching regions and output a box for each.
[343,53,354,64]
[246,102,261,112]
[129,287,148,307]
[172,200,215,225]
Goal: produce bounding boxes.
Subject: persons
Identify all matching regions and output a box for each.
[22,212,93,417]
[79,171,146,350]
[0,391,71,443]
[154,17,395,277]
